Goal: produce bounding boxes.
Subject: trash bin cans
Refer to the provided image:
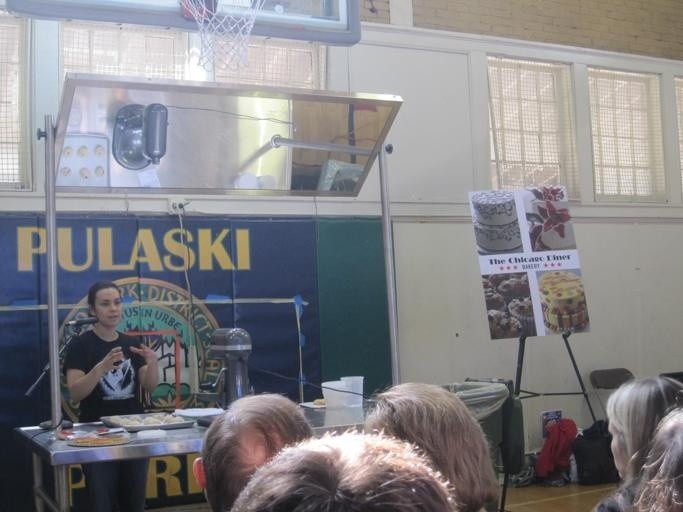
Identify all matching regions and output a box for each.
[438,381,510,487]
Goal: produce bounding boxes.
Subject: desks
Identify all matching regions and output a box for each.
[9,396,397,510]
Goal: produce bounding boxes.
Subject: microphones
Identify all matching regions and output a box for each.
[68,317,99,326]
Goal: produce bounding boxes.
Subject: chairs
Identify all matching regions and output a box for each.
[589,368,643,425]
[659,371,683,408]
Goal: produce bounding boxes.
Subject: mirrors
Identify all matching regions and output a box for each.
[48,70,404,200]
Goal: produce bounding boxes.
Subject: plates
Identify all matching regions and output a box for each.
[39,420,74,428]
[174,408,225,416]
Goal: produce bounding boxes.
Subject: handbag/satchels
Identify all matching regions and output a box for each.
[533,415,621,485]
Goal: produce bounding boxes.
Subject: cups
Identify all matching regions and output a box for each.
[320,376,365,409]
[237,174,277,190]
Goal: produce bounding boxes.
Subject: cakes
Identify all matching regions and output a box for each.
[531,189,574,249]
[472,190,522,251]
[538,271,589,333]
[482,272,534,339]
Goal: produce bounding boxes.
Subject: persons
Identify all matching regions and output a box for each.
[62,279,159,512]
[634,408,682,512]
[230,430,457,512]
[592,376,682,512]
[363,381,500,512]
[193,394,313,511]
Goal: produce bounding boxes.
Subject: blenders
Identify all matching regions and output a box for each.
[195,326,254,428]
[113,102,170,174]
